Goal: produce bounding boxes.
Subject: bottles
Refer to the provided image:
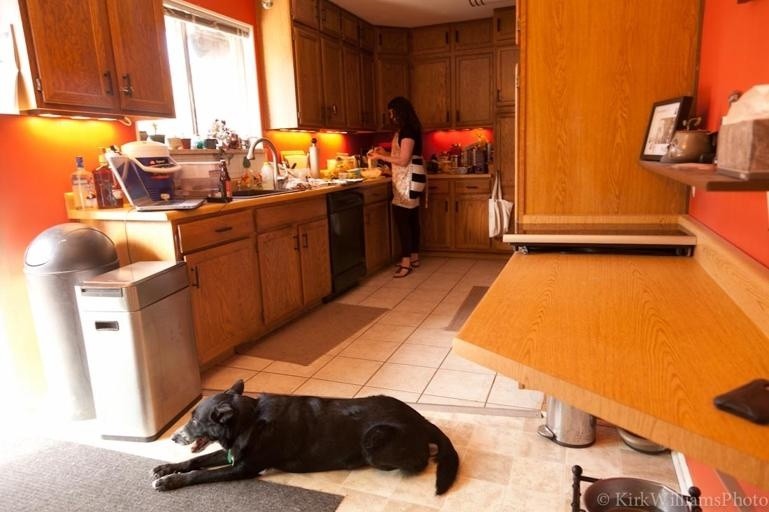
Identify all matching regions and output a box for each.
[219,161,233,203]
[431,142,485,174]
[261,162,277,190]
[668,131,714,163]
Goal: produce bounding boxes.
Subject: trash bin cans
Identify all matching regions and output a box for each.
[23,223,120,421]
[73,261,203,442]
[537,397,596,448]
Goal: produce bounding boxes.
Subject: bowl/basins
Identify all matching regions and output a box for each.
[362,171,381,178]
[584,477,690,511]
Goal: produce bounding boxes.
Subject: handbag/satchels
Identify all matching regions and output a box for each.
[489,171,514,239]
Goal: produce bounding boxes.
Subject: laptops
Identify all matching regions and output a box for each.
[106,154,205,210]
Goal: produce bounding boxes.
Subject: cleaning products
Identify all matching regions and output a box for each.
[261,160,274,190]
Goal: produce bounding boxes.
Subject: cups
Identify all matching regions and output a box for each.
[348,168,362,179]
[240,176,253,190]
[338,172,347,180]
[168,137,217,150]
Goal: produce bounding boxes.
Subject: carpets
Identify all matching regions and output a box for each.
[1,434,348,512]
[444,286,488,332]
[235,302,392,366]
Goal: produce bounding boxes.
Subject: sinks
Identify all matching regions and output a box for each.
[231,188,282,198]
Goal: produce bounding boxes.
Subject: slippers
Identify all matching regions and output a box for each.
[396,259,420,268]
[393,265,412,278]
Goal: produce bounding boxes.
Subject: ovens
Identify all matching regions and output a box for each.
[327,191,367,293]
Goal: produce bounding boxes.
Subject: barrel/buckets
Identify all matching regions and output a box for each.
[121,141,182,200]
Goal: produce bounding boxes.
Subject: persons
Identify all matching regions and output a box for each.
[367,95,429,278]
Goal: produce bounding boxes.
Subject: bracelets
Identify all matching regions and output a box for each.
[384,156,386,163]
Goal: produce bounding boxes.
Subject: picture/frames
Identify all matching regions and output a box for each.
[639,95,694,161]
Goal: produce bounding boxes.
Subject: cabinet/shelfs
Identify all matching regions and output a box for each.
[264,0,342,131]
[364,182,401,277]
[342,9,377,134]
[409,20,495,130]
[3,1,180,121]
[415,177,493,259]
[494,6,514,109]
[494,108,515,259]
[375,26,409,131]
[70,199,264,376]
[253,192,332,337]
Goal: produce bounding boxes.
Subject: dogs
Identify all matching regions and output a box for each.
[149,378,460,498]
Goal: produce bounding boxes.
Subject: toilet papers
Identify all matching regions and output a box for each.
[309,145,319,178]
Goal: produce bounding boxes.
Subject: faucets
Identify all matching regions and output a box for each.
[246,137,289,190]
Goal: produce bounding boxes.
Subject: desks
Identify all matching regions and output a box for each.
[451,248,769,490]
